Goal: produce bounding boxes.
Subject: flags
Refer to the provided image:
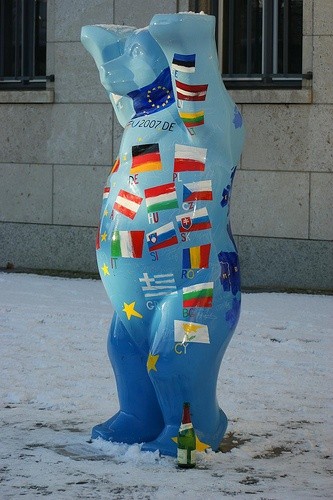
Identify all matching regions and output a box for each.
[110,231,145,258]
[138,271,179,301]
[173,142,209,173]
[182,179,215,202]
[175,205,215,233]
[128,143,162,175]
[177,111,209,129]
[145,222,179,252]
[173,319,212,346]
[107,157,121,176]
[127,65,175,120]
[143,183,180,214]
[181,243,211,272]
[112,188,143,221]
[181,280,215,311]
[95,187,110,249]
[173,80,210,104]
[171,51,197,76]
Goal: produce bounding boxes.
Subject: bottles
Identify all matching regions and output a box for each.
[176,402,197,469]
